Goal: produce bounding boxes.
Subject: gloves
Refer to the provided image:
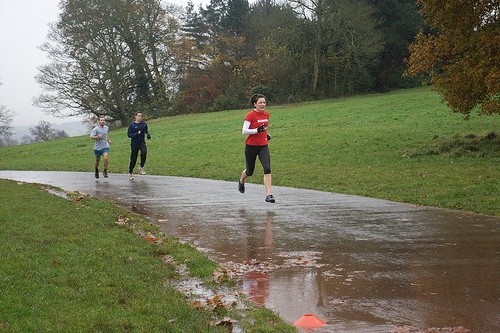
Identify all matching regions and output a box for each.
[267,134,271,140]
[136,130,143,135]
[147,134,151,140]
[257,124,266,132]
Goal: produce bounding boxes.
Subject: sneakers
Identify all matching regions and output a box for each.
[238,178,245,193]
[265,195,275,203]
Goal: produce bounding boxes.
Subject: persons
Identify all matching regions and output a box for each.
[238,93,276,203]
[127,111,151,180]
[89,115,112,179]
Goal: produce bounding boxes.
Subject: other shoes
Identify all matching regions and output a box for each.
[103,169,108,177]
[138,166,146,175]
[128,173,134,180]
[95,168,99,179]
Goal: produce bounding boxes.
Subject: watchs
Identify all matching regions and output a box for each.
[98,135,99,139]
